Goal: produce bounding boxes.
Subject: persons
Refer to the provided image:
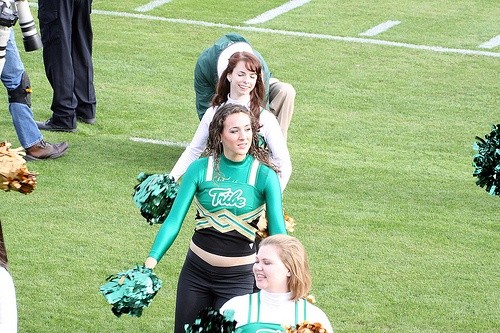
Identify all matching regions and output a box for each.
[98,103,289,333]
[0,141,39,333]
[0,0,69,160]
[35,0,97,132]
[131,51,296,239]
[183,234,333,333]
[194,33,296,143]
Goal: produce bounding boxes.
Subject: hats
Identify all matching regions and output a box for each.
[218,42,253,83]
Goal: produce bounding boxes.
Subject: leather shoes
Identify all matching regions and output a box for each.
[77,114,97,125]
[35,119,76,133]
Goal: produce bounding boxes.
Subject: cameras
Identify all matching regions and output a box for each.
[15,0,43,52]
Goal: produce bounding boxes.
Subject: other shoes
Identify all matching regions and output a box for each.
[23,139,69,161]
